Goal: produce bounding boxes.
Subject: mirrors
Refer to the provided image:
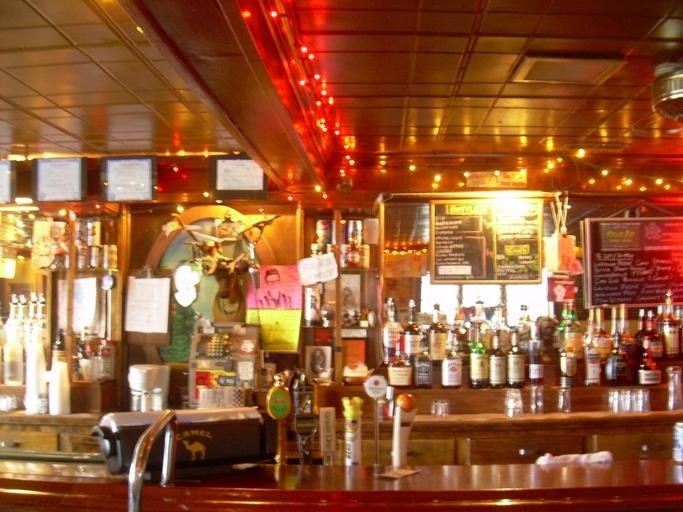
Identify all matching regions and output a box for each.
[383,195,682,352]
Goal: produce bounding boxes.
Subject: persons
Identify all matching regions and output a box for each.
[258,267,294,308]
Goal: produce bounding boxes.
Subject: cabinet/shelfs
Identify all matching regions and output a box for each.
[595,410,683,459]
[465,418,588,465]
[301,193,382,472]
[326,418,461,477]
[0,202,132,462]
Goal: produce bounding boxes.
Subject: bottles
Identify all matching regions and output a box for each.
[256,366,317,420]
[670,422,683,465]
[0,290,111,416]
[673,464,683,485]
[375,291,682,385]
[307,218,370,271]
[307,296,335,329]
[382,238,427,253]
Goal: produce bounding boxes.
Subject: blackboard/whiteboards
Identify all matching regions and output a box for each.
[584,217,683,309]
[430,198,543,284]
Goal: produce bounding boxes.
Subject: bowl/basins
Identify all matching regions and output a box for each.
[343,375,364,386]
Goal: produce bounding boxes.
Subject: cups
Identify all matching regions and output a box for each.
[606,389,651,414]
[556,389,571,413]
[665,365,681,409]
[431,398,448,417]
[529,386,543,414]
[504,389,523,417]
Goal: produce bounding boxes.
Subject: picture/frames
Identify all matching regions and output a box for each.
[209,155,267,199]
[31,156,88,202]
[100,156,157,201]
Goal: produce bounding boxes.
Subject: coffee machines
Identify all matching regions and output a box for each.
[127,363,170,412]
